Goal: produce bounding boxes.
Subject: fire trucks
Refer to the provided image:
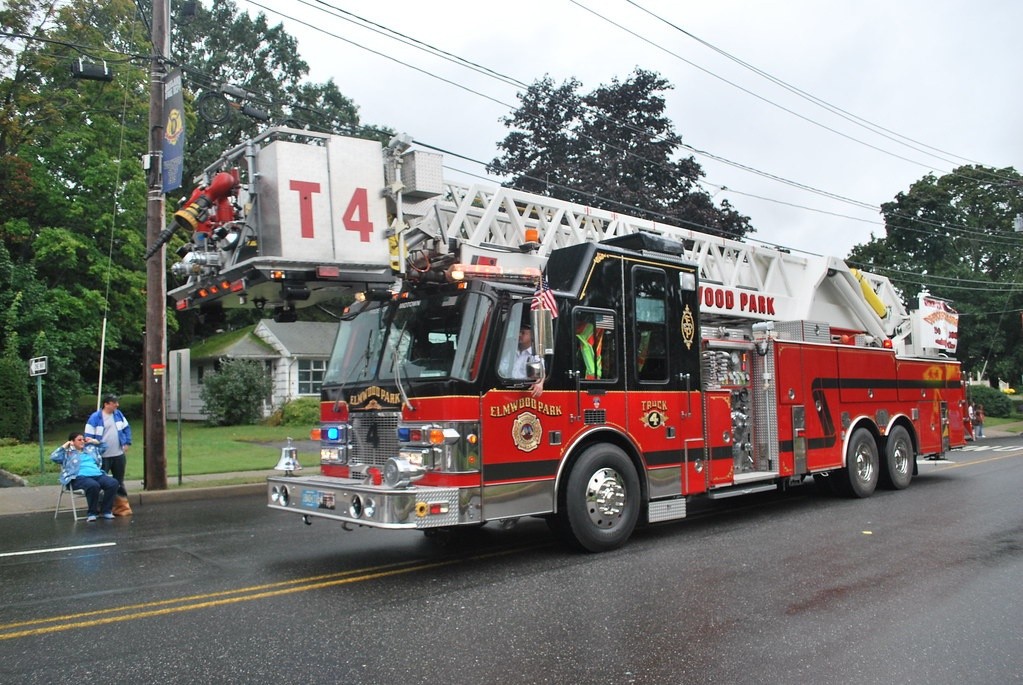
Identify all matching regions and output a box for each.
[145,126,975,556]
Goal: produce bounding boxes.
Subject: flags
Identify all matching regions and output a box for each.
[531,274,559,320]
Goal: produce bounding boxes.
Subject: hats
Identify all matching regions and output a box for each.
[521,321,533,329]
[102,393,121,403]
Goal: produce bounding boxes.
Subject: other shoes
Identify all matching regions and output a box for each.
[87,515,96,521]
[99,513,114,518]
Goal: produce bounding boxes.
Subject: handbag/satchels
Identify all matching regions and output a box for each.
[110,487,133,516]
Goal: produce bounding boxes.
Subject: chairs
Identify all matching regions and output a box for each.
[54,464,121,522]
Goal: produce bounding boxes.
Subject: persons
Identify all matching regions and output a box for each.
[498,323,545,398]
[84,394,132,497]
[968,401,975,435]
[50,432,119,522]
[976,404,986,437]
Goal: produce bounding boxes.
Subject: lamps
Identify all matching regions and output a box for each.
[250,296,269,310]
[237,293,248,305]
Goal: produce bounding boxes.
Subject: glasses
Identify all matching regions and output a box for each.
[77,438,86,441]
